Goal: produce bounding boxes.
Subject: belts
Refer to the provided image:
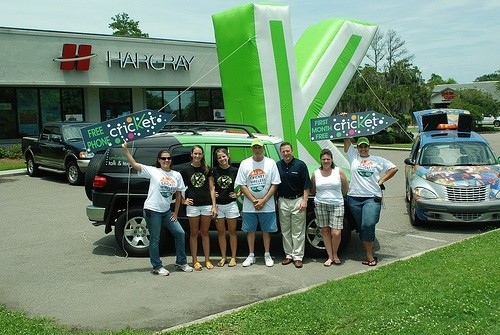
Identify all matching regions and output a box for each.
[279,192,303,200]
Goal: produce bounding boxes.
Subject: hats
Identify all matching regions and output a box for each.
[356,137,369,146]
[251,138,264,147]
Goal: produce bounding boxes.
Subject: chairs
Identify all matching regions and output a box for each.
[458,144,480,163]
[426,147,444,164]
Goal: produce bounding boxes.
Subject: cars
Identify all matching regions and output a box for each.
[474,113,500,128]
[404,108,500,226]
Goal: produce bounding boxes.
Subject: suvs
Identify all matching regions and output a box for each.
[84,122,361,258]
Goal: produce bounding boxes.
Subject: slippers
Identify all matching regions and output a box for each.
[368,257,377,266]
[362,259,369,265]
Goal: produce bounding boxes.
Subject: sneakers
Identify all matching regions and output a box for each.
[174,263,194,273]
[243,255,256,267]
[264,254,274,267]
[154,266,169,275]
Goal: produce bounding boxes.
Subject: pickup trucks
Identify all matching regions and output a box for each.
[21,122,94,186]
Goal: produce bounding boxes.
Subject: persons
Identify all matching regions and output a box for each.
[310,149,348,266]
[239,140,278,267]
[121,141,193,276]
[339,112,398,265]
[210,148,243,267]
[276,142,310,268]
[181,145,217,269]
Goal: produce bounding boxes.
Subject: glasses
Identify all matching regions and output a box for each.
[159,157,171,160]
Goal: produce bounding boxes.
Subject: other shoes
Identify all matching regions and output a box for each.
[228,257,236,266]
[282,258,293,265]
[293,259,303,268]
[205,260,214,270]
[324,258,333,267]
[333,257,341,264]
[216,257,226,266]
[194,261,203,271]
